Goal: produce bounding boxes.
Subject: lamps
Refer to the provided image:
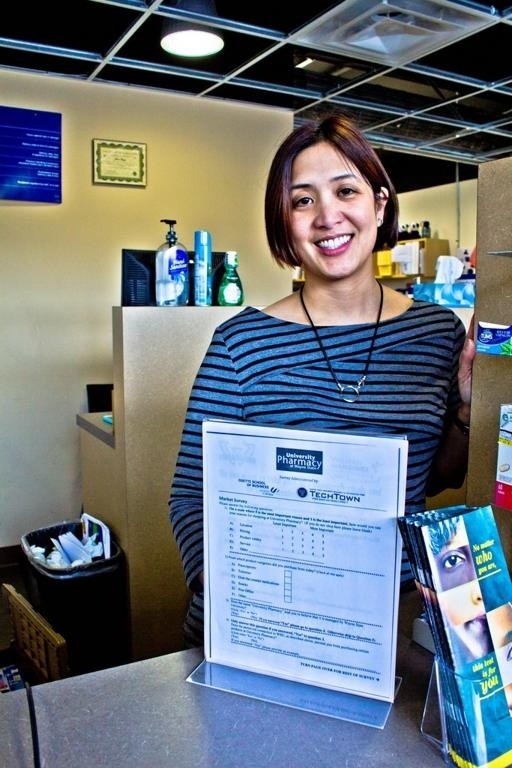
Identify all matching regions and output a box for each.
[159,1,226,59]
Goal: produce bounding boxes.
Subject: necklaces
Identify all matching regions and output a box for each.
[299,277,383,403]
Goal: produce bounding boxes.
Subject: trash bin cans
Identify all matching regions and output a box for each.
[20,520,126,675]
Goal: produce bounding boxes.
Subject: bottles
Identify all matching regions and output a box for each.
[192,229,243,308]
[421,219,432,238]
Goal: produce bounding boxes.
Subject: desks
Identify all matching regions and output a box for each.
[32,628,454,768]
[2,685,32,766]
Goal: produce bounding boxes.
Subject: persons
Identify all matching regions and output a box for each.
[486,601,512,721]
[421,515,494,666]
[167,116,475,649]
